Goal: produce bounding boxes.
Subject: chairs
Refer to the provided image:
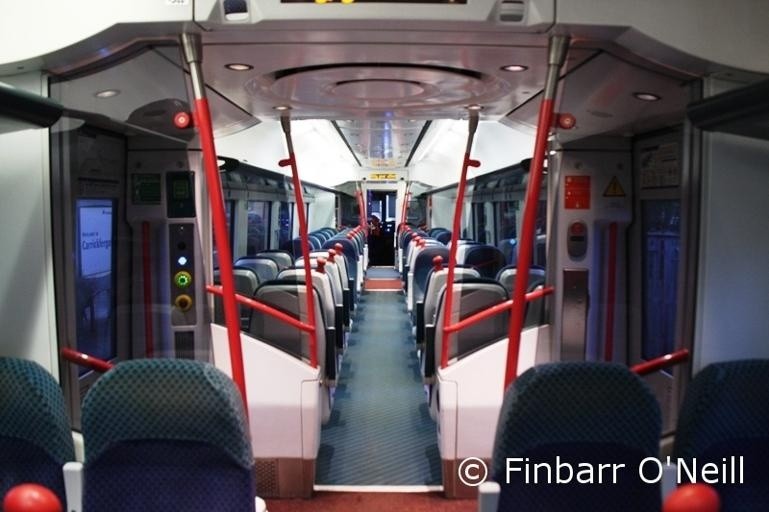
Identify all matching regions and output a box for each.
[399,227,769,512]
[0,227,369,512]
